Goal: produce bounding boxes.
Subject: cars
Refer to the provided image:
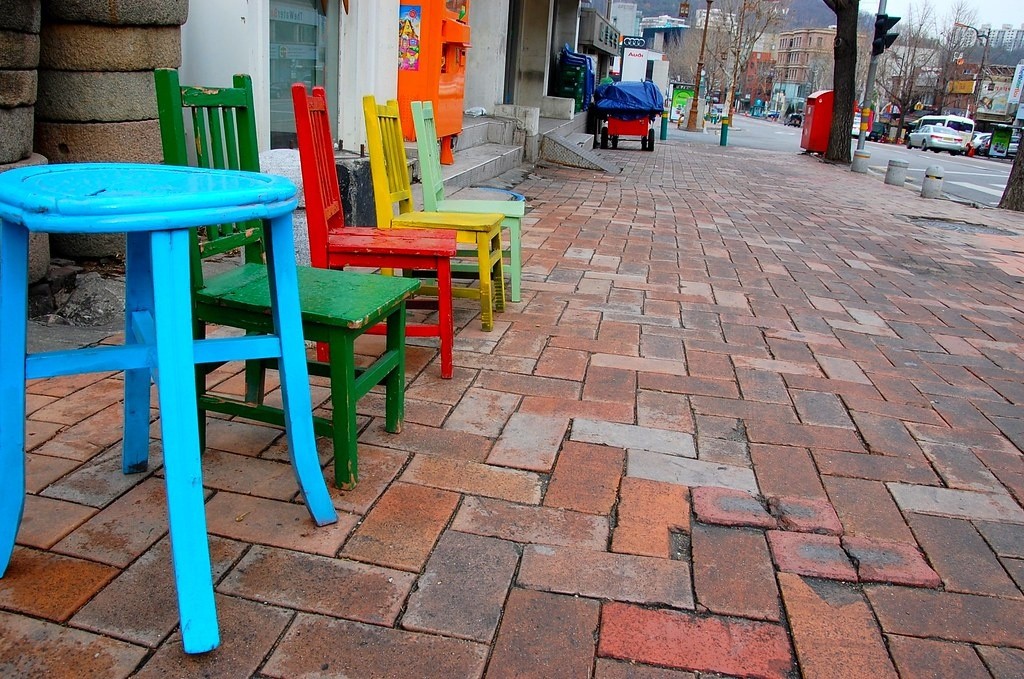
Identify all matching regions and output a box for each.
[852,117,870,138]
[784,114,802,128]
[764,110,780,118]
[906,123,962,156]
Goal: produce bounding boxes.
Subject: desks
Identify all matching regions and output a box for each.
[0,161,341,655]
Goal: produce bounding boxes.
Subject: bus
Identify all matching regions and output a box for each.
[915,115,974,154]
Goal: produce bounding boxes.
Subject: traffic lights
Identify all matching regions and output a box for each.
[872,13,902,56]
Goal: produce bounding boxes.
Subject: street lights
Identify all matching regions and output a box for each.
[955,22,992,119]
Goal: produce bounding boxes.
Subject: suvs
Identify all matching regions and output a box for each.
[979,136,1021,154]
[974,133,993,156]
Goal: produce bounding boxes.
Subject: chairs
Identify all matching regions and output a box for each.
[559,41,595,110]
[411,100,525,302]
[361,94,508,332]
[153,65,422,492]
[291,81,458,381]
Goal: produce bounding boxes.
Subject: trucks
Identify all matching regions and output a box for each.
[867,122,884,141]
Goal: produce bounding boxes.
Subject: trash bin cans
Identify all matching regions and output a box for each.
[753,106,762,117]
[801,89,857,151]
